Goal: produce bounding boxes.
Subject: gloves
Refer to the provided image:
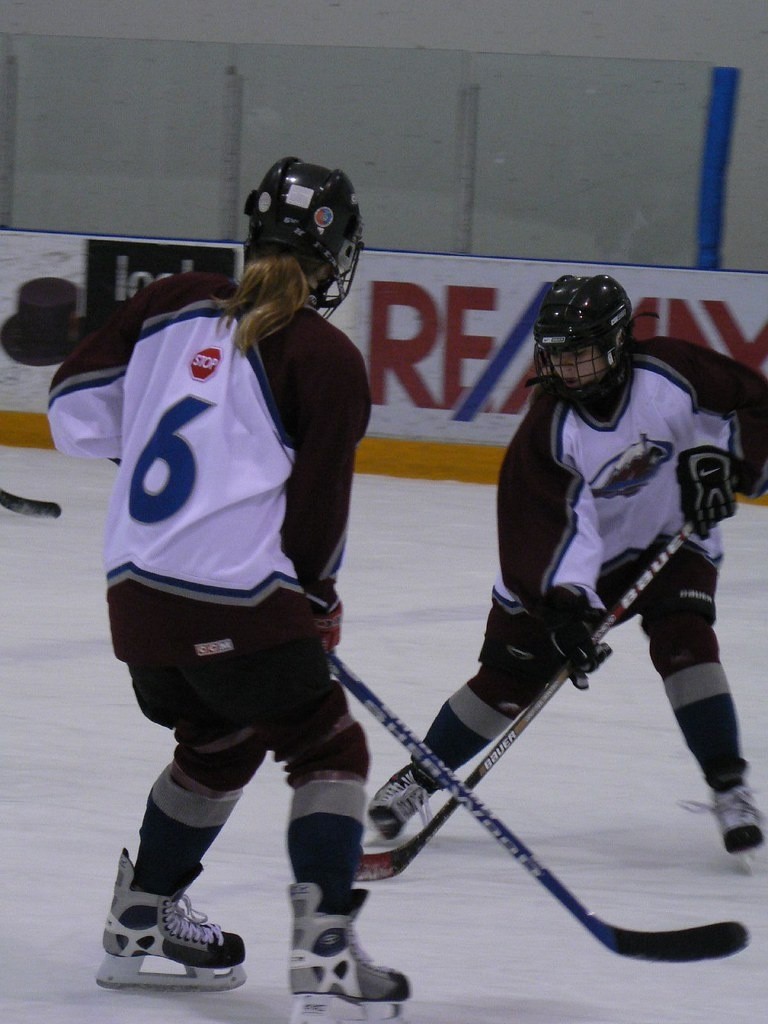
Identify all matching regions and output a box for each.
[676,445,736,541]
[305,592,342,654]
[539,600,612,690]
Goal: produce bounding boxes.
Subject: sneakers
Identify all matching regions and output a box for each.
[360,764,440,847]
[289,883,409,1024]
[677,787,766,876]
[95,848,247,992]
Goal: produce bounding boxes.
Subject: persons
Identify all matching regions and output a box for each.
[44,157,412,1002]
[367,274,768,854]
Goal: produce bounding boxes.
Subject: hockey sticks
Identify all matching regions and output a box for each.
[349,519,696,883]
[321,648,751,965]
[1,486,62,520]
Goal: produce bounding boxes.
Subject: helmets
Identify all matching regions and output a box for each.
[242,156,364,320]
[534,275,631,403]
